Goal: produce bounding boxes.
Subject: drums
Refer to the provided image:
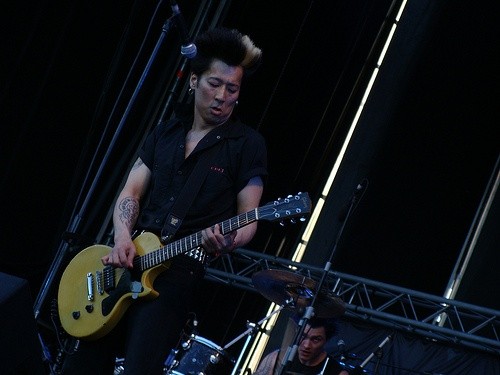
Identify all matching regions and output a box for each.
[114,360,124,375]
[163,333,240,375]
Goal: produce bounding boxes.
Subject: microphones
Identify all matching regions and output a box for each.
[170,0,198,58]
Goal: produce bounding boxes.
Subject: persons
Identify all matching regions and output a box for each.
[254,315,349,375]
[63,29,268,375]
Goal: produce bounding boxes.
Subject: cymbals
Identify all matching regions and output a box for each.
[252,268,346,315]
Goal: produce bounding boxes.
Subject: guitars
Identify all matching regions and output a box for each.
[57,191,313,339]
